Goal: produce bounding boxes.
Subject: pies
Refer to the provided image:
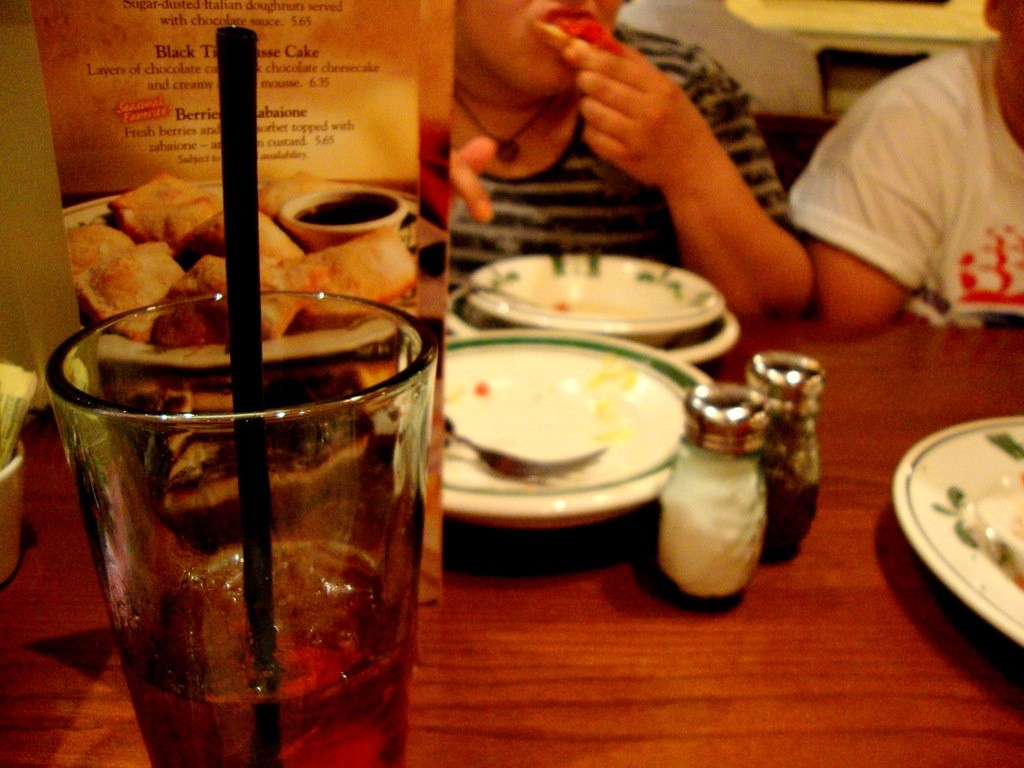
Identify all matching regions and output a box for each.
[60,174,325,348]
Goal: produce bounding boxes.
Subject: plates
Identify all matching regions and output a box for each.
[444,288,739,363]
[59,183,420,364]
[891,415,1024,648]
[442,332,713,527]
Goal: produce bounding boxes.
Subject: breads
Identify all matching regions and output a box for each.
[533,21,574,49]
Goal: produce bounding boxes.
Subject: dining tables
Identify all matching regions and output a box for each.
[1,315,1024,766]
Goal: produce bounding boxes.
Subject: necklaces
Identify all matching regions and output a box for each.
[453,93,556,162]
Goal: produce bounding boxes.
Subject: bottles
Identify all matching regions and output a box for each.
[658,384,767,601]
[745,350,828,564]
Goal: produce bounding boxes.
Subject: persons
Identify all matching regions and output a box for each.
[789,0,1024,327]
[444,0,813,317]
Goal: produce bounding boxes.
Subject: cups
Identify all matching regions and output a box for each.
[43,288,439,767]
[0,440,24,586]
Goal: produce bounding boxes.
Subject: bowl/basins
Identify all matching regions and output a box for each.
[277,188,406,252]
[465,253,726,351]
